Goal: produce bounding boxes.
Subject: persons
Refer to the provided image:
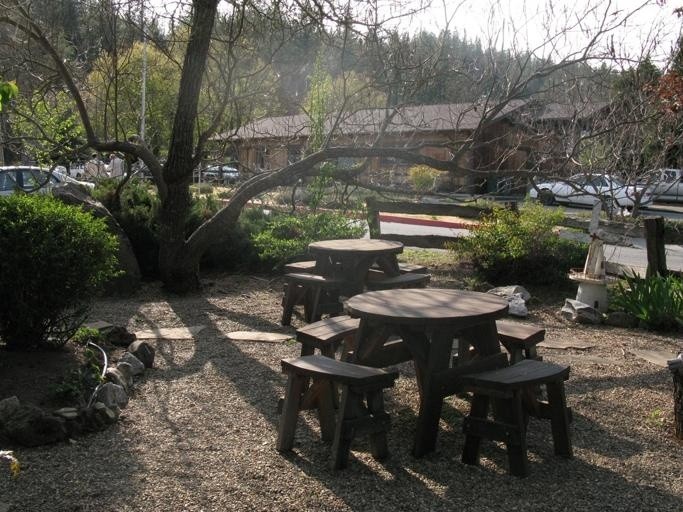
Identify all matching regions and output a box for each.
[105,153,122,178]
[84,154,104,177]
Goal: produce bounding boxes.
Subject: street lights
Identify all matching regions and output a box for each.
[140,17,158,185]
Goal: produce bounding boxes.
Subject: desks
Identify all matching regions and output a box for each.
[340,288,510,461]
[306,238,404,276]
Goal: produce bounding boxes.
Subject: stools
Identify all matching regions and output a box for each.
[496,319,546,364]
[276,354,395,469]
[463,358,571,476]
[282,258,432,325]
[296,315,361,359]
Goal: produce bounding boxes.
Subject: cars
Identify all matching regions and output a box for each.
[201,165,239,182]
[529,173,653,211]
[0,165,95,196]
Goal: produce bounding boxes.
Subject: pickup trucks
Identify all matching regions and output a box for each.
[636,168,683,200]
